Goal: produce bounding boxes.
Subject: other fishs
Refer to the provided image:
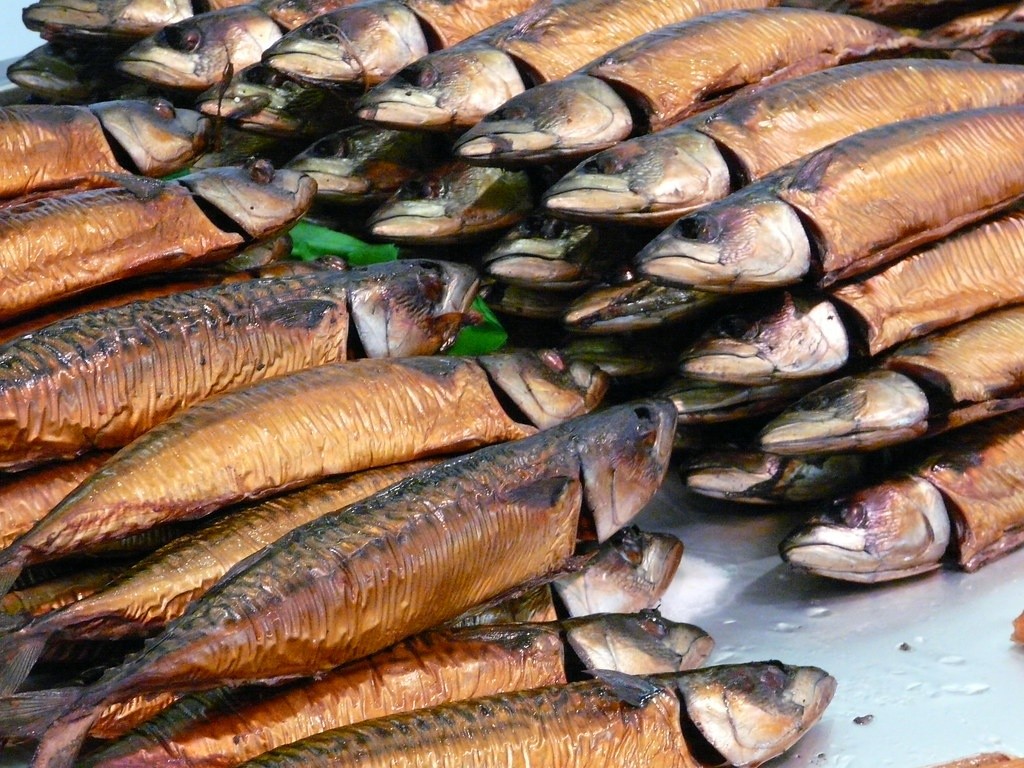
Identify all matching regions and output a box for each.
[0,0,1024,768]
